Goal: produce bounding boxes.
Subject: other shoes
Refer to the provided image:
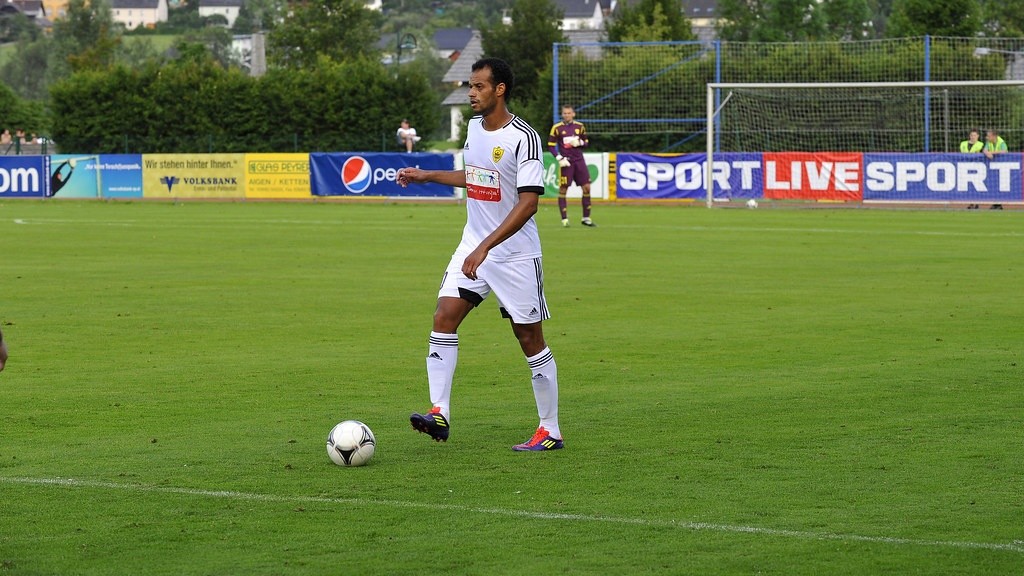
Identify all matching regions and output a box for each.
[412,136,421,142]
[967,204,979,209]
[990,204,1003,209]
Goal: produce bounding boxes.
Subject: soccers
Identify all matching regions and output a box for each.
[326,420,376,466]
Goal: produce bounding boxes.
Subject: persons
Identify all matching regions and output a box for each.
[392,57,570,454]
[395,117,422,154]
[959,129,984,209]
[548,104,598,228]
[1,128,42,145]
[982,129,1009,210]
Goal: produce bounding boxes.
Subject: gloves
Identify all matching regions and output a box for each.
[556,155,571,167]
[571,138,584,147]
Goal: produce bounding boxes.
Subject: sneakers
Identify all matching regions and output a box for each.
[562,219,569,227]
[410,407,450,442]
[512,426,564,451]
[581,217,597,227]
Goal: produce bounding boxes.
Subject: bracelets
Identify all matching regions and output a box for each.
[558,156,564,161]
